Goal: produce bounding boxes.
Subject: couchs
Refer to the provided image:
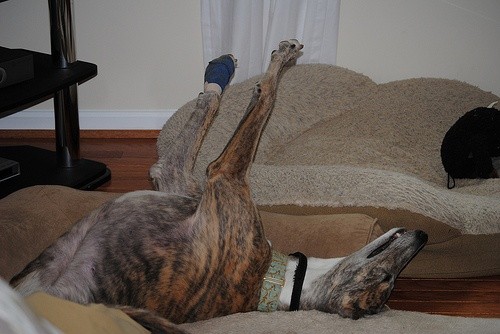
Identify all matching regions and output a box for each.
[157,63,500,279]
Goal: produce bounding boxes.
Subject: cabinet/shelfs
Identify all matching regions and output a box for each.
[0,0,111,190]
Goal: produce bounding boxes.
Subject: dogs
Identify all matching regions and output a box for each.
[8,39,429,333]
